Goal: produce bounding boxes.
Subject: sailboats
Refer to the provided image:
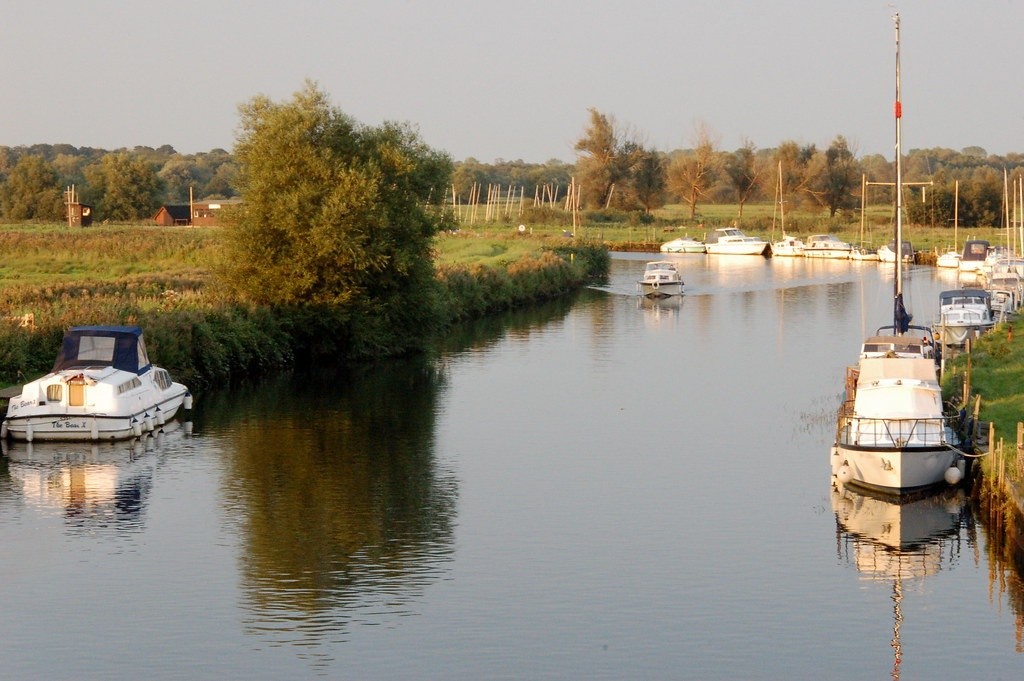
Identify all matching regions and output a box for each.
[827,474,967,681]
[876,166,1024,350]
[769,160,807,256]
[828,9,961,496]
[848,175,879,263]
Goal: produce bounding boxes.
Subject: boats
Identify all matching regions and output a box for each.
[639,261,686,299]
[704,228,770,255]
[659,235,706,253]
[802,235,852,259]
[0,327,194,445]
